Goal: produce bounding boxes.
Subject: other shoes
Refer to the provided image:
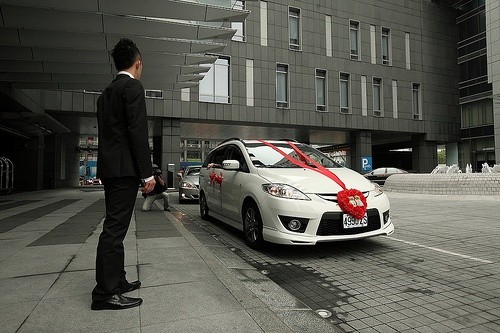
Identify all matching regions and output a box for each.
[164,207,171,211]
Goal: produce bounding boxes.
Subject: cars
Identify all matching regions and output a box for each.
[176,165,204,203]
[362,166,408,185]
[198,137,394,246]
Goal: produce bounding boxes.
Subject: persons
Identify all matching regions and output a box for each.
[142,169,173,212]
[91,37,156,310]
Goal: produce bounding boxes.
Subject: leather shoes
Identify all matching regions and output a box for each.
[91,294,143,310]
[116,280,142,294]
